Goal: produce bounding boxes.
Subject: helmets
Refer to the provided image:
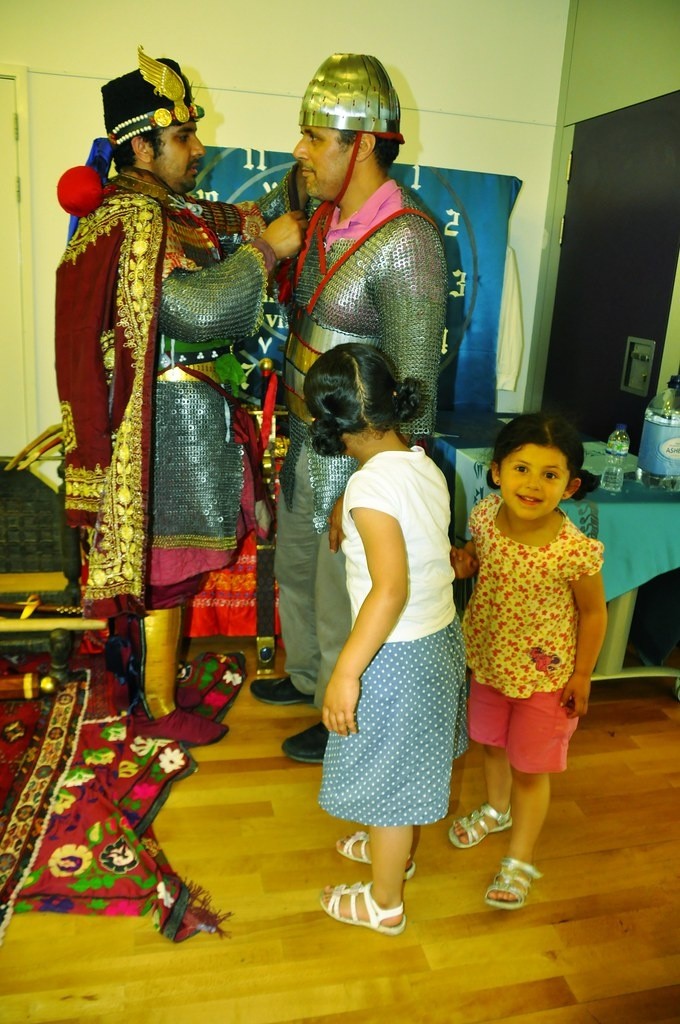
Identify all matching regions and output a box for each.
[299,52,401,134]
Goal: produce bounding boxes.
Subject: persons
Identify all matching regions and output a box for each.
[57,43,310,746]
[302,341,470,934]
[248,52,448,764]
[443,412,610,910]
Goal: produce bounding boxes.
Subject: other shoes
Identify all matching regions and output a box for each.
[250,675,315,705]
[281,721,329,764]
[135,706,229,745]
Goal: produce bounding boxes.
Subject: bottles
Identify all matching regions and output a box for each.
[635,373,680,491]
[599,424,630,491]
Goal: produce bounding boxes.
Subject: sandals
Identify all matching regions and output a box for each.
[319,881,407,935]
[483,857,544,909]
[448,802,512,848]
[336,831,416,880]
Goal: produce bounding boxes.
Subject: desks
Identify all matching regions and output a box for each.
[428,409,680,702]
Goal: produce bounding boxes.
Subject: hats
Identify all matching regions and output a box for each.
[100,46,204,146]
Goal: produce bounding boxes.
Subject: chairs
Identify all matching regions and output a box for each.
[0,409,292,686]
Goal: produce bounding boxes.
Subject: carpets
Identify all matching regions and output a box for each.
[0,647,246,944]
